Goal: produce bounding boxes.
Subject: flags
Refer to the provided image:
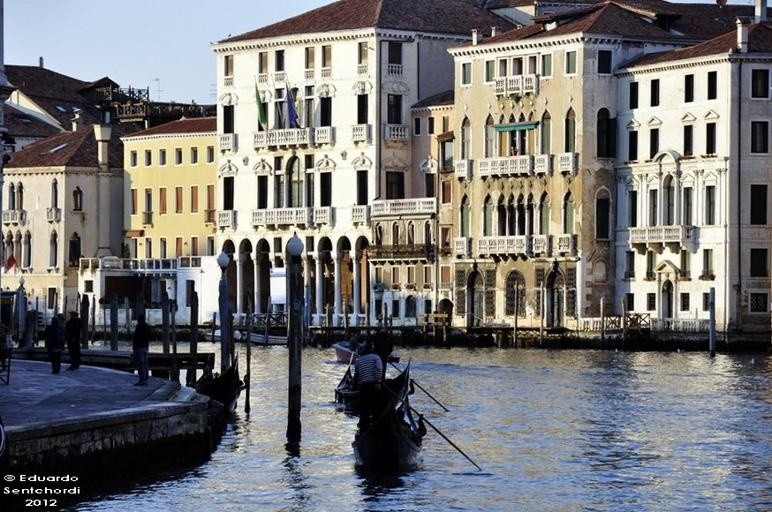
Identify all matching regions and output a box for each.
[4,254,19,272]
[253,79,266,124]
[285,85,300,129]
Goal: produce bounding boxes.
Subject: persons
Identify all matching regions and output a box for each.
[43,312,82,375]
[348,325,393,395]
[132,314,153,388]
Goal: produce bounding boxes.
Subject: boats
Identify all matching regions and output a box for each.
[244,330,288,347]
[204,329,221,343]
[333,343,400,365]
[336,357,414,417]
[194,351,242,415]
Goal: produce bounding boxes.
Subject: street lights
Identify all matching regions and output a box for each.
[215,250,232,379]
[285,230,308,419]
